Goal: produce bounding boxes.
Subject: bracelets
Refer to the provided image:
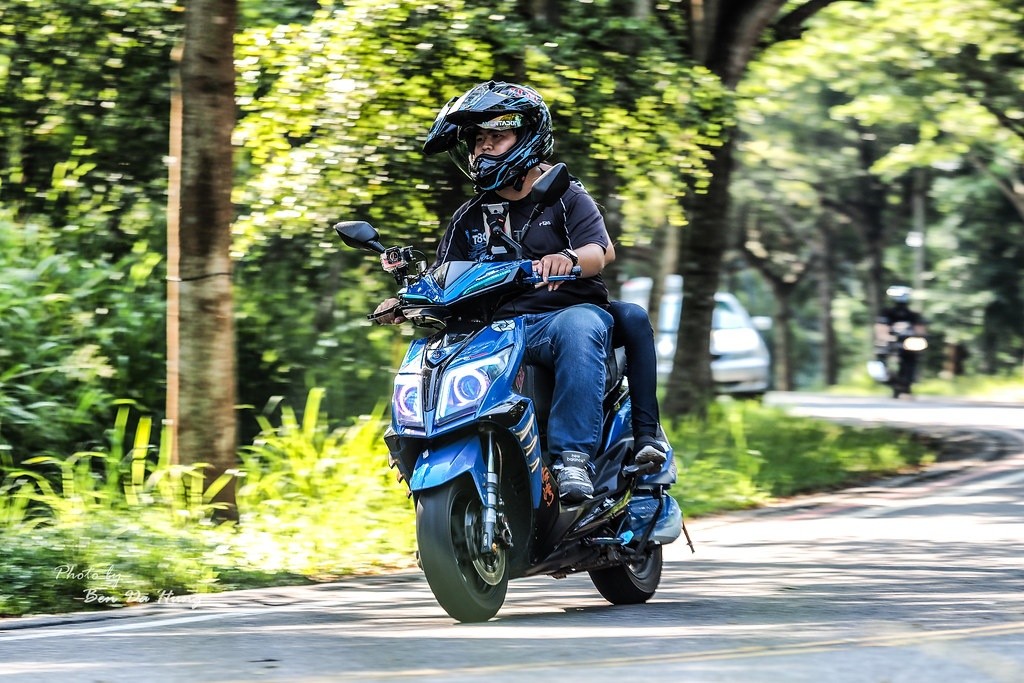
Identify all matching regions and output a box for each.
[557,252,569,258]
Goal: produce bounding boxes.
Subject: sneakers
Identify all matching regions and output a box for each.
[634,435,668,463]
[552,450,594,503]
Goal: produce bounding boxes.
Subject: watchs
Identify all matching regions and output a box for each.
[558,249,578,267]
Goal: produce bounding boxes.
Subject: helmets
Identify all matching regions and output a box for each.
[422,80,554,195]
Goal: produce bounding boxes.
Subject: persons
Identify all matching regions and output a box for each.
[372,81,665,503]
[875,284,927,384]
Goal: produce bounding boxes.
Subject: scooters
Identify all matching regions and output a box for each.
[331,219,697,623]
[864,317,932,397]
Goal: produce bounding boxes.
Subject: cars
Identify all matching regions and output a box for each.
[618,271,683,308]
[630,288,773,402]
